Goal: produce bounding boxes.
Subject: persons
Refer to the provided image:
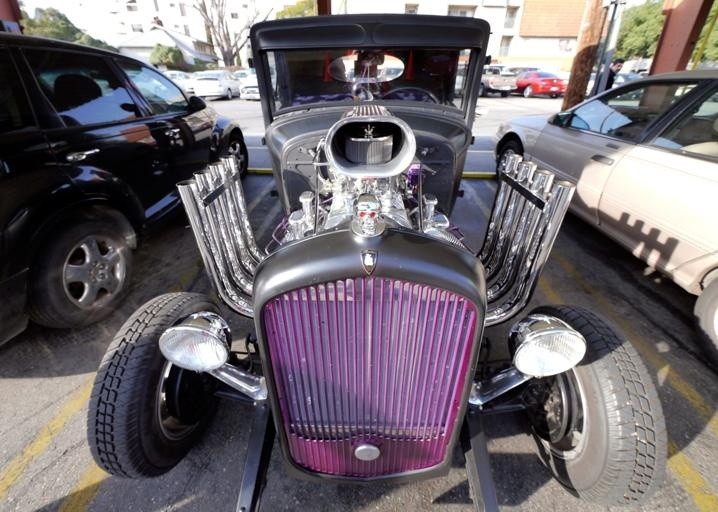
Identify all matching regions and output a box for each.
[586,58,625,100]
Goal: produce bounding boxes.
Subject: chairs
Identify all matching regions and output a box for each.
[679,117,718,158]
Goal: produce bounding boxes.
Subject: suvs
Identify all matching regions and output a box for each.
[0,32,246,350]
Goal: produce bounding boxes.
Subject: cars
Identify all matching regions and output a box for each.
[88,13,670,505]
[454,63,646,100]
[162,65,268,101]
[496,71,717,358]
